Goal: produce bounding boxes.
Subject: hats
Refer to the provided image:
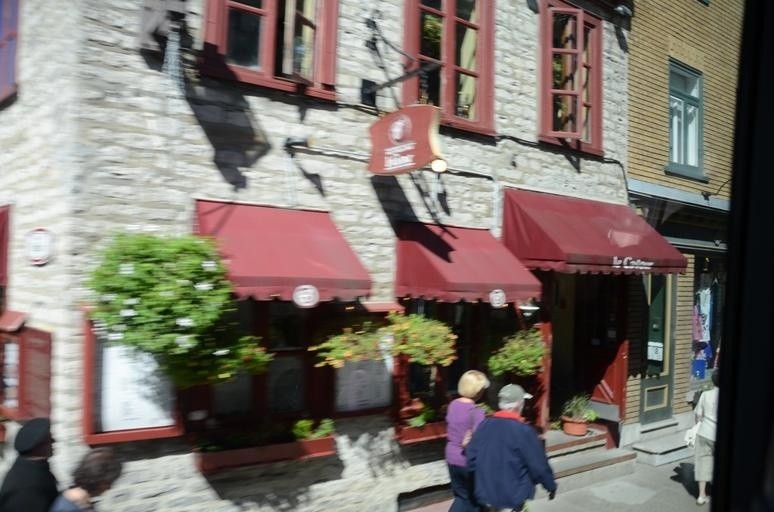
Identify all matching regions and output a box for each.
[13,418,50,453]
[498,383,532,403]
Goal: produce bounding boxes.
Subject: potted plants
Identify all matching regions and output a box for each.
[559,390,597,436]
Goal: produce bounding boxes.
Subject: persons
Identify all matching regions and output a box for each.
[445,370,490,512]
[466,383,558,512]
[0,416,58,512]
[693,368,721,505]
[48,449,122,512]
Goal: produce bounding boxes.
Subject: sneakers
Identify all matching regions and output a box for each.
[697,495,710,505]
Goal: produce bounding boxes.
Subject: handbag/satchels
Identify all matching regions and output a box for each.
[684,422,700,447]
